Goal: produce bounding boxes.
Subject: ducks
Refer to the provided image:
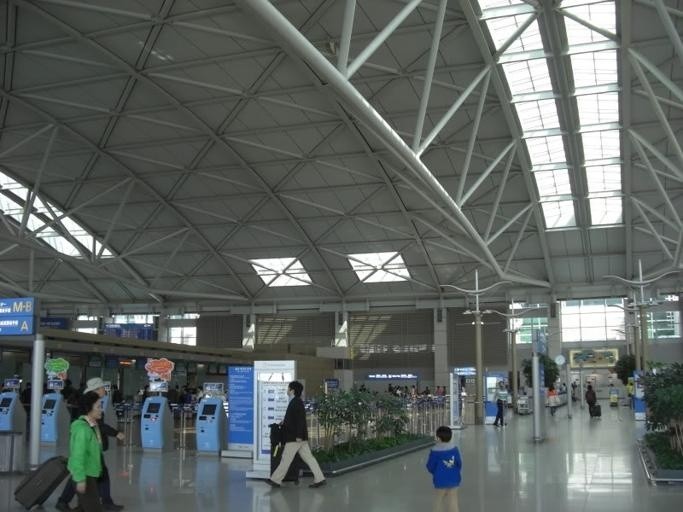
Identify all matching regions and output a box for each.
[591,404,601,416]
[14,456,69,511]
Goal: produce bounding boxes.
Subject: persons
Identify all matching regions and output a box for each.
[0,376,205,512]
[547,380,596,417]
[425,426,462,512]
[263,381,327,489]
[492,381,507,427]
[608,384,619,406]
[627,382,634,409]
[358,382,447,407]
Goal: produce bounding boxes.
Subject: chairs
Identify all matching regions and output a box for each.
[56,503,71,512]
[102,504,123,512]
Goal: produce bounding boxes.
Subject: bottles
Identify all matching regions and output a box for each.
[84,377,108,394]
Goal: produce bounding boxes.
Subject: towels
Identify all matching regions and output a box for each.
[0,430,23,475]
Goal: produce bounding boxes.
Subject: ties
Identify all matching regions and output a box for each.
[146,402,160,414]
[0,397,13,409]
[43,398,57,411]
[202,404,217,416]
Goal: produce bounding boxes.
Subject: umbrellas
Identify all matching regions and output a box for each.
[309,479,326,488]
[265,479,280,488]
